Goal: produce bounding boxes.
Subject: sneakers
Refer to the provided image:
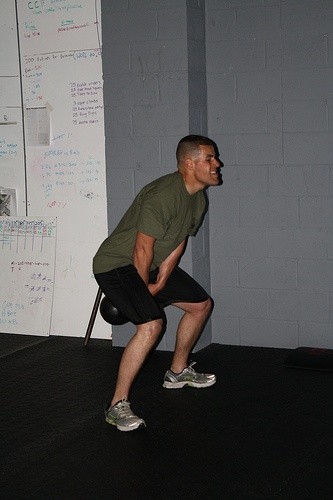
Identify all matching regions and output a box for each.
[104,395,147,432]
[163,362,216,389]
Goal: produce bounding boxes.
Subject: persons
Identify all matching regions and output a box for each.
[92,135,221,433]
